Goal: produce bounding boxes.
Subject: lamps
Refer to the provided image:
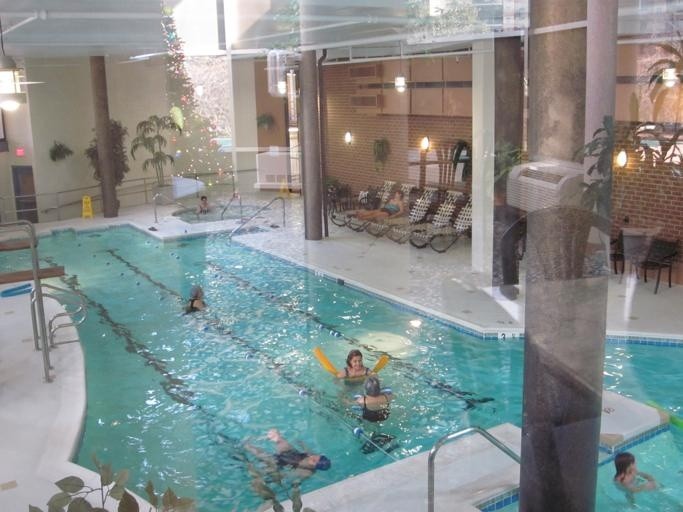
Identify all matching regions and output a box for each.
[277,69,286,95]
[0,26,27,114]
[393,42,406,93]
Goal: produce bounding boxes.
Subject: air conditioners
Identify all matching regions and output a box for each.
[347,65,379,81]
[348,93,381,110]
[502,159,584,219]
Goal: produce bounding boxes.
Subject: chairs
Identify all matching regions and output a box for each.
[330,177,473,252]
[610,227,646,274]
[633,235,680,294]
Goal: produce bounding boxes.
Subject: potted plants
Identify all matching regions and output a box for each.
[129,112,184,206]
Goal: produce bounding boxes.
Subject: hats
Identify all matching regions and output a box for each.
[190,286,201,298]
[314,456,331,470]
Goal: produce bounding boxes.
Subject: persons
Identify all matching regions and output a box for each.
[345,375,394,425]
[356,190,403,221]
[246,426,330,484]
[196,196,214,215]
[614,453,656,495]
[335,350,372,389]
[185,286,208,321]
